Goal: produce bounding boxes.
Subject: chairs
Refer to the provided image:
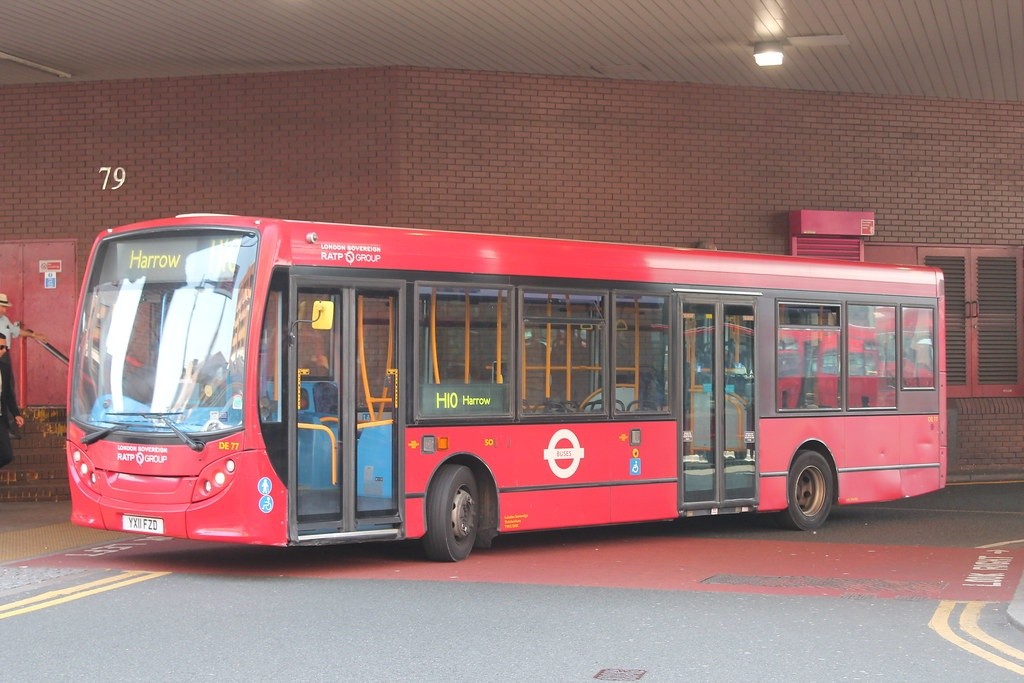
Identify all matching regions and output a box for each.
[520,396,661,417]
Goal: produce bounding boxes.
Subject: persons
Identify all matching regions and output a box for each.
[0,293,48,439]
[0,333,24,469]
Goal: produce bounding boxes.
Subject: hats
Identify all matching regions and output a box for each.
[0,294,12,307]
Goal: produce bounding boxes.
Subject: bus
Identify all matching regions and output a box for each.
[65,212,948,563]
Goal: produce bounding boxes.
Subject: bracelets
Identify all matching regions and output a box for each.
[31,333,36,338]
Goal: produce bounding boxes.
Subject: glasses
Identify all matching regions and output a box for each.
[0,345,8,350]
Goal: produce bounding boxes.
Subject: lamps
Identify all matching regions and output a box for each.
[753,43,784,66]
